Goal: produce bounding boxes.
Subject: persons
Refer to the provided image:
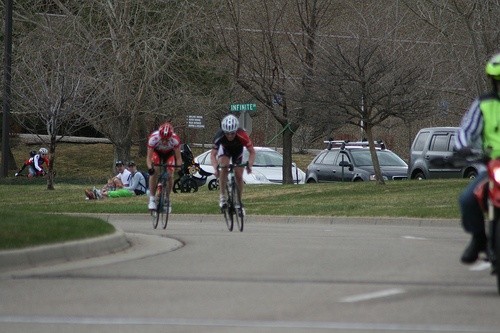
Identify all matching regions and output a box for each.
[15,147,50,178]
[454,54,500,263]
[210,114,256,217]
[145,122,182,213]
[84,161,145,199]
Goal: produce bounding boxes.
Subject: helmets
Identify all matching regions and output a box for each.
[159,122,173,139]
[221,114,239,133]
[39,148,48,154]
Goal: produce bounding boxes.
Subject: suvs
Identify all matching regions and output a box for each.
[409,127,488,180]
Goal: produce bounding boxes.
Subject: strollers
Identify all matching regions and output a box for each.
[172,141,219,193]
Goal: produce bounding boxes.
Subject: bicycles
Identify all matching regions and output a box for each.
[216,161,249,232]
[150,161,182,230]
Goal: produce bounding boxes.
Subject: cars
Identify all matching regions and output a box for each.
[307,138,409,182]
[192,146,307,185]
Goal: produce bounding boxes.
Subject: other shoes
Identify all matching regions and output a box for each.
[164,200,172,213]
[219,199,227,208]
[148,202,157,211]
[460,235,488,263]
[92,185,102,200]
[236,207,245,217]
[84,189,92,199]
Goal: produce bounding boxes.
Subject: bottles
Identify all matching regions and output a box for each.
[229,185,232,198]
[157,183,162,196]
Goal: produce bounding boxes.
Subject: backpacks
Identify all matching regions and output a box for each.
[133,171,149,190]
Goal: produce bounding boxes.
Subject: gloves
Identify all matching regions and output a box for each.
[177,170,184,178]
[148,167,155,175]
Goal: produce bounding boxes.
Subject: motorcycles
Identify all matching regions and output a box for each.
[473,159,500,296]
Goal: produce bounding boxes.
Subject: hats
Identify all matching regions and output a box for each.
[127,161,136,166]
[116,160,124,166]
[486,52,500,80]
